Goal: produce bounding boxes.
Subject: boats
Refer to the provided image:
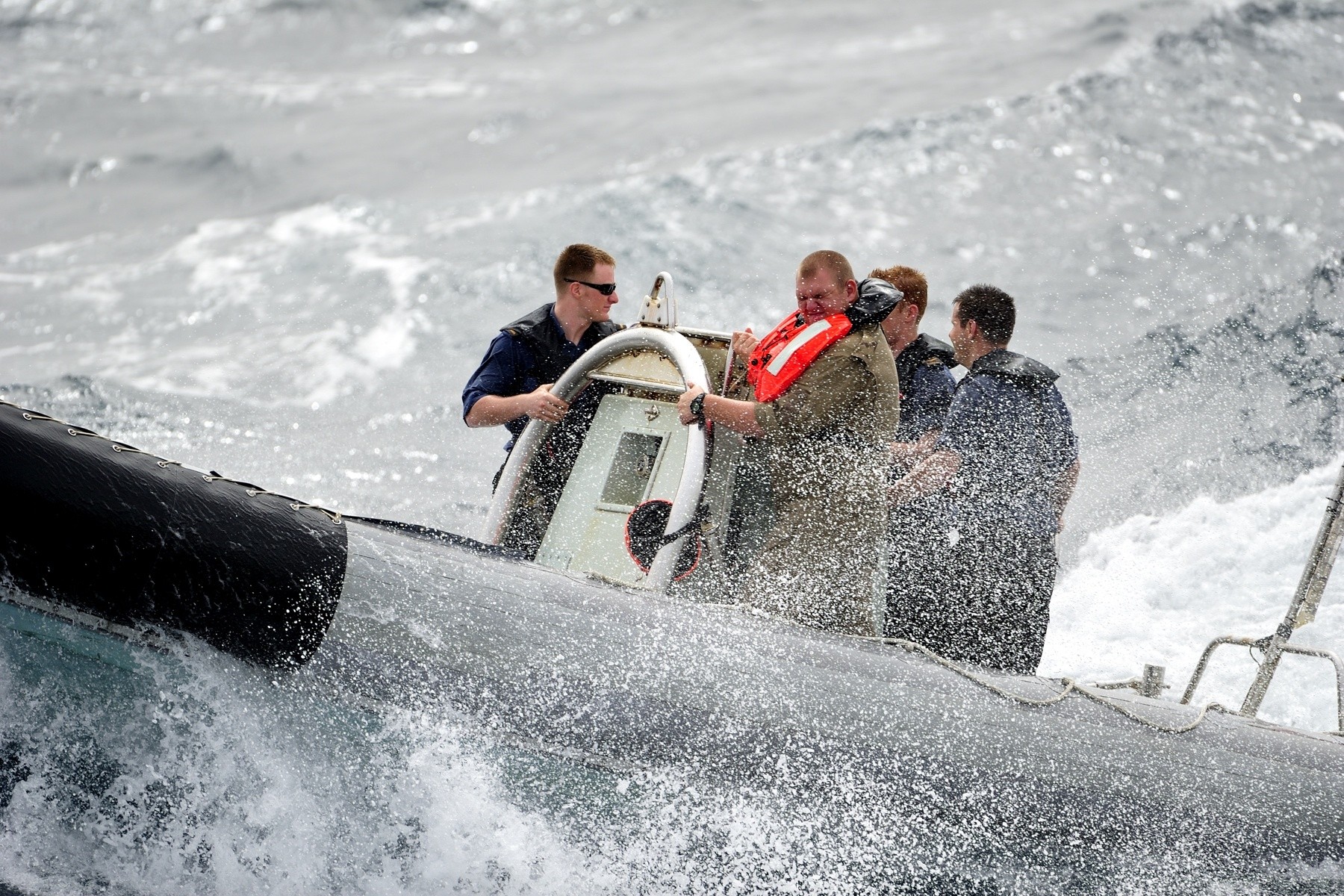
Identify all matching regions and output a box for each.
[0,269,1343,896]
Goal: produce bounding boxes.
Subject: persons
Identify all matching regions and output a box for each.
[676,251,899,640]
[864,268,1078,674]
[462,244,627,562]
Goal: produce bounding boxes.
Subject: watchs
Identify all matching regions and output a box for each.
[689,392,708,431]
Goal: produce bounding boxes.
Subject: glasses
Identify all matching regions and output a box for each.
[564,278,616,295]
